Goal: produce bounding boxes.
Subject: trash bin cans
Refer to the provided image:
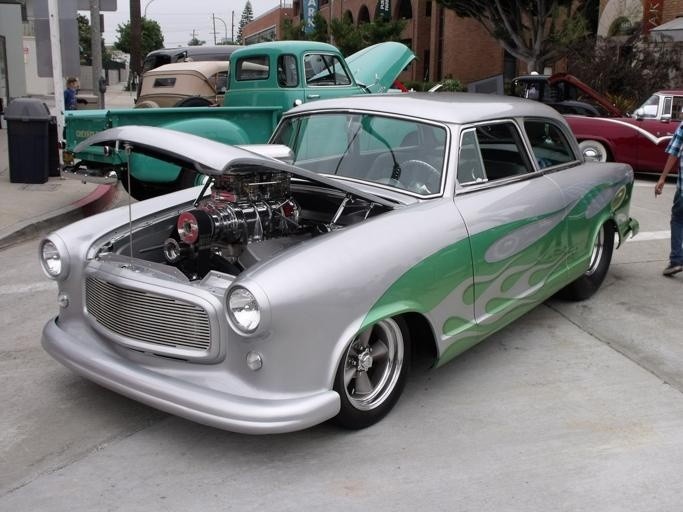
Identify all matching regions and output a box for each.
[7,98,49,183]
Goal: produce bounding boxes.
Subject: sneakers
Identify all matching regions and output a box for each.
[664,264,683,275]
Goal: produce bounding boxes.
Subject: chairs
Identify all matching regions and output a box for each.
[460,159,527,181]
[367,152,436,183]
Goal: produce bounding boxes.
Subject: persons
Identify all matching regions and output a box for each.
[654,120,682,277]
[62,76,88,149]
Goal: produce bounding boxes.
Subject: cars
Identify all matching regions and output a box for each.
[38,90,640,439]
[548,73,683,179]
[134,58,283,108]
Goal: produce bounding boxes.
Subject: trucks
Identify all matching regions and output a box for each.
[62,38,425,203]
